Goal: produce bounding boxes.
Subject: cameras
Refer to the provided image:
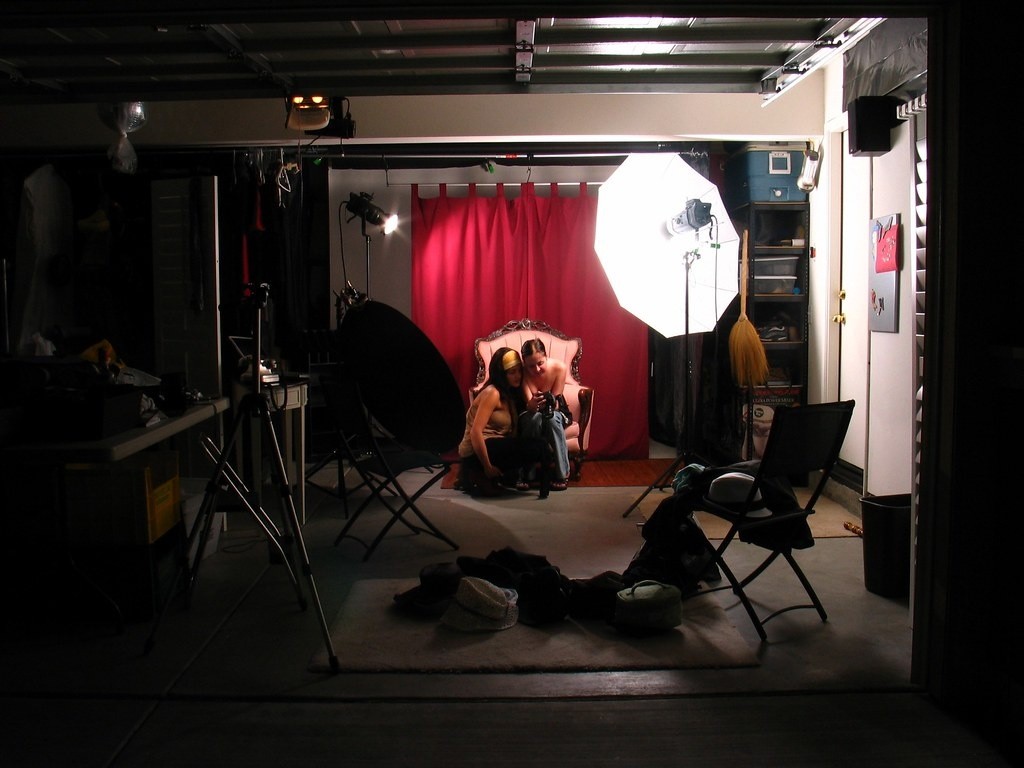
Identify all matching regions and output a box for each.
[540,391,555,419]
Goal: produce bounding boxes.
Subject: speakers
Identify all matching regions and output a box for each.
[848,96,890,155]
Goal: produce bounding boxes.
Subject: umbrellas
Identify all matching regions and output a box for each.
[595,153,739,340]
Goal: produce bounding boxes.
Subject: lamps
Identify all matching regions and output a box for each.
[796,142,820,192]
[813,36,842,49]
[282,92,329,130]
[345,190,397,235]
[781,62,808,75]
[759,78,781,95]
[304,95,356,139]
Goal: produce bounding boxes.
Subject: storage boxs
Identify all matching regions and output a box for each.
[860,494,911,603]
[179,476,224,569]
[739,256,799,275]
[740,384,804,423]
[738,275,797,294]
[120,447,181,544]
[722,142,806,209]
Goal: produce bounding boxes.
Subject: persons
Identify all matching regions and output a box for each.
[457,339,574,493]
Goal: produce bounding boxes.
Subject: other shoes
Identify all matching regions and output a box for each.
[475,479,506,497]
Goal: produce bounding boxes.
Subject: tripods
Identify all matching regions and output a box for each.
[142,305,343,675]
[622,291,718,518]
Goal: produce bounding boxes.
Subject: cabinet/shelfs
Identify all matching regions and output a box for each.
[726,201,810,459]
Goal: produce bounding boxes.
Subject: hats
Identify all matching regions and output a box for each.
[440,576,519,632]
[703,471,773,518]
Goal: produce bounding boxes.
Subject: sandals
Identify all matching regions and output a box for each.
[550,478,568,491]
[515,480,532,491]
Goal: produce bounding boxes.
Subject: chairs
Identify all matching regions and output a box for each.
[692,399,855,642]
[469,321,593,478]
[315,365,463,556]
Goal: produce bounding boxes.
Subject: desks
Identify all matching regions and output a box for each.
[76,397,230,461]
[232,381,309,530]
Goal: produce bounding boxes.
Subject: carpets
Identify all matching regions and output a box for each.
[306,480,769,673]
[441,457,674,490]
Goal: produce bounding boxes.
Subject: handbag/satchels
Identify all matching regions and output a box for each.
[615,579,683,636]
